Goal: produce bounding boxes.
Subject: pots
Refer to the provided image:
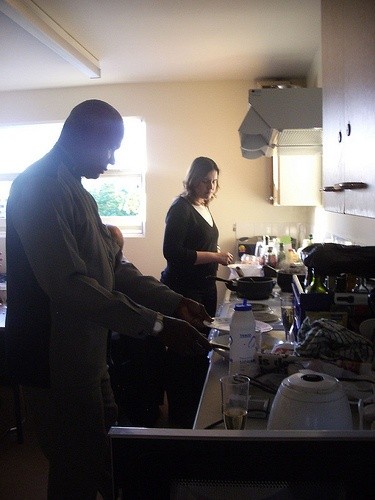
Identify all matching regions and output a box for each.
[205,275,276,300]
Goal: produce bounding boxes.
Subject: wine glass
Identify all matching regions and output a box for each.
[279,293,295,344]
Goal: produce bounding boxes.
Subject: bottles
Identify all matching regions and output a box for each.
[304,267,370,294]
[263,247,276,276]
[228,304,257,380]
[277,243,286,264]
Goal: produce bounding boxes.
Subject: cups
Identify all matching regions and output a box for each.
[220,375,250,430]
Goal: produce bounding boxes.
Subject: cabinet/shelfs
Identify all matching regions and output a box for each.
[321,0,375,219]
[272,146,321,207]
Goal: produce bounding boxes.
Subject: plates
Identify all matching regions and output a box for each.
[204,316,273,334]
[211,335,284,360]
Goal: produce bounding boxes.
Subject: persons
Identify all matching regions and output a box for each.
[159,157,234,429]
[5,98,215,500]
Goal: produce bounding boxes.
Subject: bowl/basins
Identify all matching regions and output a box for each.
[247,303,279,322]
[277,269,307,291]
[228,264,263,279]
